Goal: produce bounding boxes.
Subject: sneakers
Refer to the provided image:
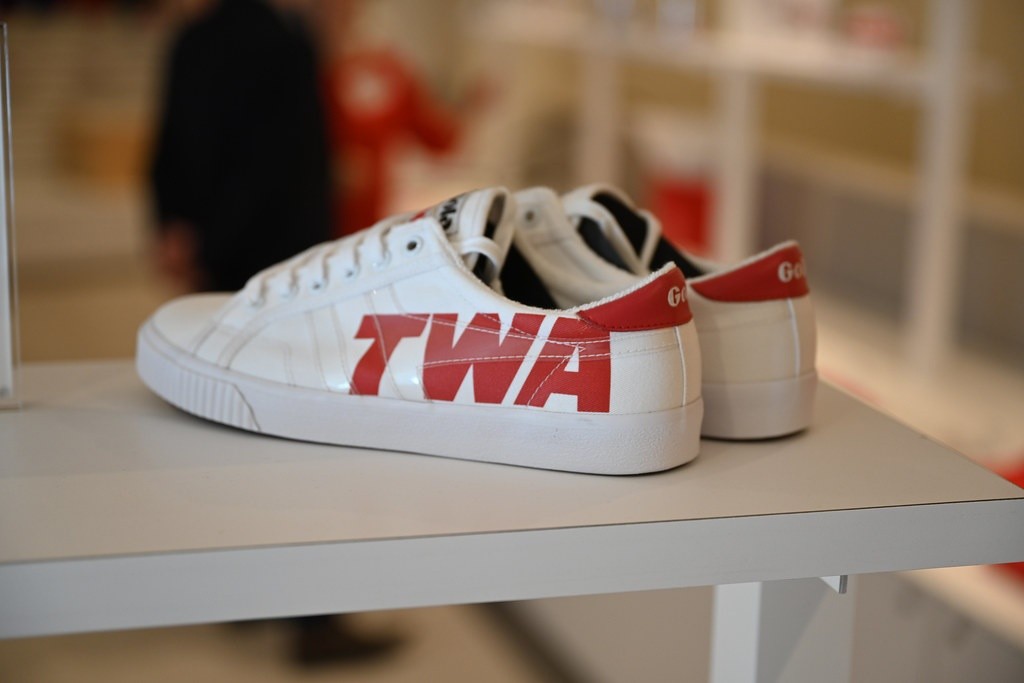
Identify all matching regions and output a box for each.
[513,183,818,442]
[134,184,707,472]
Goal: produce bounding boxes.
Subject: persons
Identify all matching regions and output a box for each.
[144,0,457,668]
